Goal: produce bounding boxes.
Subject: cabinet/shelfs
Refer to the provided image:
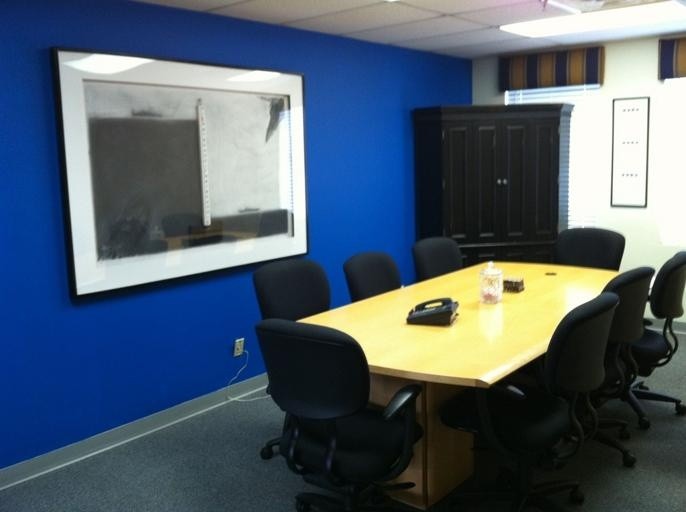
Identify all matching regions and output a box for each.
[412,104,561,266]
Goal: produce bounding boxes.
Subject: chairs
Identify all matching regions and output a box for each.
[550,226,625,271]
[411,235,463,282]
[254,259,331,322]
[255,318,424,511]
[108,208,288,259]
[343,251,402,303]
[584,267,654,469]
[439,290,619,511]
[619,251,685,430]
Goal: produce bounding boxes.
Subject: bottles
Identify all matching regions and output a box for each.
[478,262,501,304]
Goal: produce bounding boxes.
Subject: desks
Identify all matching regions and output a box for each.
[294,260,622,512]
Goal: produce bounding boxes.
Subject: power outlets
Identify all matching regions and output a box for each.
[233,339,246,359]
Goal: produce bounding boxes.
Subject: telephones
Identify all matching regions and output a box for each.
[407,298,459,326]
